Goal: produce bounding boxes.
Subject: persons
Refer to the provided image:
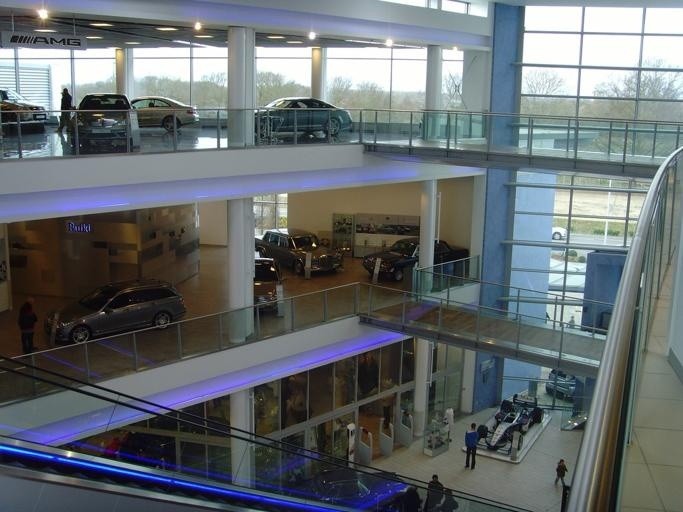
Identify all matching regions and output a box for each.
[18,294,39,355]
[464,422,479,470]
[555,459,568,486]
[54,88,72,133]
[566,315,576,328]
[406,473,458,511]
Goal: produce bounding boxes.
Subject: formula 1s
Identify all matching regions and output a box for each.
[477,393,544,460]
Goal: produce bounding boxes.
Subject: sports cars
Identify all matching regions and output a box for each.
[299,467,420,512]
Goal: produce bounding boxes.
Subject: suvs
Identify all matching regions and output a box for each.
[44,278,187,347]
[545,370,576,400]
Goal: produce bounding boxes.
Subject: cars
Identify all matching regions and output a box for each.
[362,237,469,281]
[69,93,138,152]
[0,87,50,132]
[130,96,200,131]
[255,227,344,274]
[255,257,282,312]
[552,226,567,240]
[254,96,352,136]
